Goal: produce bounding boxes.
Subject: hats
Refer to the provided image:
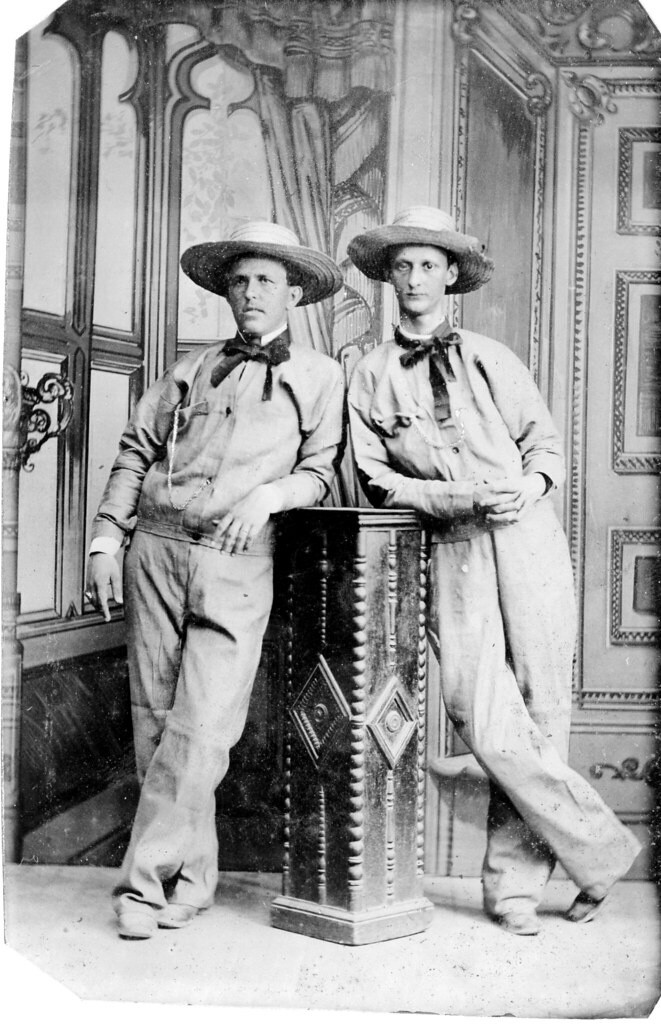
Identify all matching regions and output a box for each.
[348,206,494,294]
[180,222,344,307]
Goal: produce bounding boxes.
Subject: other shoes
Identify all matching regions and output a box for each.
[567,883,617,924]
[485,902,541,936]
[157,902,207,926]
[118,908,158,938]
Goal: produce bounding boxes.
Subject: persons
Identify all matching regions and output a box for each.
[343,202,645,938]
[84,220,348,940]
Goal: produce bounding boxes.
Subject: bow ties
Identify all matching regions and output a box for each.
[394,321,464,421]
[211,328,291,402]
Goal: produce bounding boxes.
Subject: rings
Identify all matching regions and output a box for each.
[85,591,92,599]
